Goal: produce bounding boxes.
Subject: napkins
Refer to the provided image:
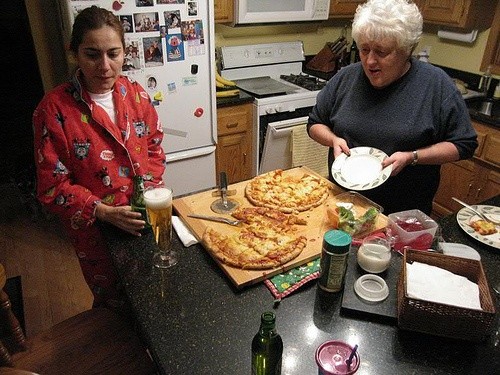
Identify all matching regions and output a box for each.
[172,216,199,247]
[406,261,483,311]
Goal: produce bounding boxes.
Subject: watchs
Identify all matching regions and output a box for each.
[411,151,418,167]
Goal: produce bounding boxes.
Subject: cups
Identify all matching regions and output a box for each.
[315,339,360,375]
[357,235,391,273]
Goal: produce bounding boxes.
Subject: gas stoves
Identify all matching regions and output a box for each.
[218,39,329,105]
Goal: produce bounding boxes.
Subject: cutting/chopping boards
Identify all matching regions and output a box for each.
[171,165,389,287]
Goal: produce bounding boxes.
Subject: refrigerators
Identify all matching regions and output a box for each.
[58,1,218,197]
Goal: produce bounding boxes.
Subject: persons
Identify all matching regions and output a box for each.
[183,24,190,40]
[129,51,139,69]
[188,2,197,16]
[306,0,479,217]
[138,14,151,31]
[32,4,166,308]
[122,17,131,31]
[149,77,156,89]
[151,19,159,31]
[166,14,179,27]
[125,45,137,56]
[144,41,161,62]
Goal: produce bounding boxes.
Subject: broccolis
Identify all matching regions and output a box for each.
[338,206,378,232]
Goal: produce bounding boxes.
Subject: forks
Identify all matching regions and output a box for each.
[187,213,246,225]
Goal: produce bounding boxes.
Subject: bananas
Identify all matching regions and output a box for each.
[216,70,236,87]
[216,89,239,97]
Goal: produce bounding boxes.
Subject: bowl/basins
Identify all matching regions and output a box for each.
[387,209,438,255]
[323,191,384,236]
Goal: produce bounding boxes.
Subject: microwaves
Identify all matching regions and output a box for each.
[230,0,330,26]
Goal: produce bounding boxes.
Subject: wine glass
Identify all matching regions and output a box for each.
[142,185,178,268]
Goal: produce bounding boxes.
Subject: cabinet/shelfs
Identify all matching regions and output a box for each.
[214,0,234,24]
[329,0,496,30]
[215,103,252,187]
[430,122,500,221]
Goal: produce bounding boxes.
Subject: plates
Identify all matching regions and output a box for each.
[457,205,500,249]
[331,146,393,190]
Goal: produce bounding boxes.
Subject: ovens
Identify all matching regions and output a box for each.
[252,103,330,175]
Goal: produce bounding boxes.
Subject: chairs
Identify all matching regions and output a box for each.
[0,264,158,375]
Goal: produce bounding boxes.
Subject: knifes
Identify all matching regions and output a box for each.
[330,36,349,55]
[219,172,227,206]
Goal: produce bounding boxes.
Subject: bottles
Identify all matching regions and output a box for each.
[317,230,352,292]
[250,311,283,375]
[350,39,359,65]
[130,175,153,234]
[477,66,492,91]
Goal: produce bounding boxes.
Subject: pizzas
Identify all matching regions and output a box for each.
[203,207,307,270]
[245,169,328,213]
[470,219,497,235]
